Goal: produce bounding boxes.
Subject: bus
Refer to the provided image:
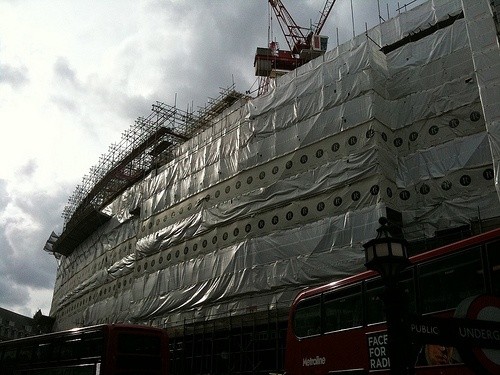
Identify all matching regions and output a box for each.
[283,229,500,375]
[0,321,170,375]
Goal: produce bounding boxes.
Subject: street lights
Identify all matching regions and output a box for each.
[363,217,416,375]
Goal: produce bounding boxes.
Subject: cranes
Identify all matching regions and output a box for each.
[251,0,336,80]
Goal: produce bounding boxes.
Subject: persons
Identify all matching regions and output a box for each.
[415,344,462,368]
